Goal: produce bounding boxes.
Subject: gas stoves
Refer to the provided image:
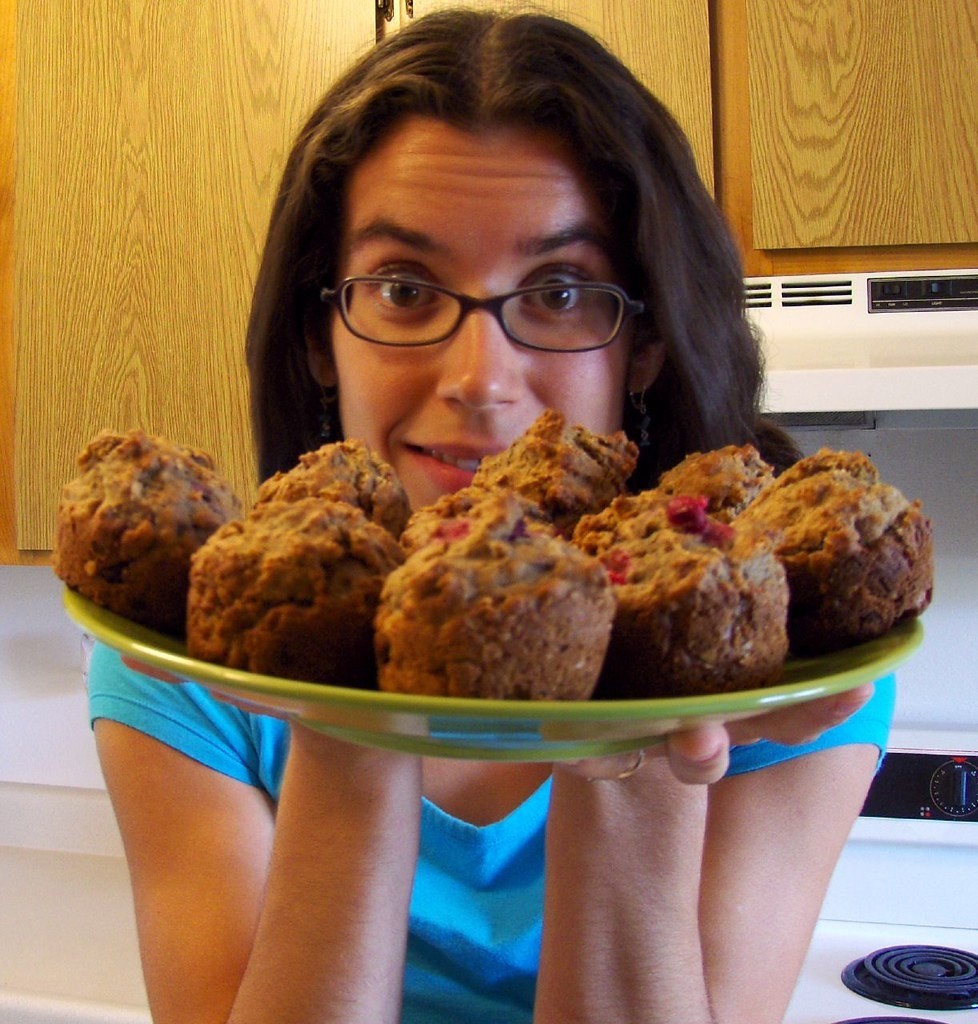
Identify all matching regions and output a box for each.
[777,746,978,1023]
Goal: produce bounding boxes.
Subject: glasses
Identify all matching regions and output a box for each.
[323,275,644,353]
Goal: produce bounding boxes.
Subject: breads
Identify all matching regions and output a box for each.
[52,410,935,702]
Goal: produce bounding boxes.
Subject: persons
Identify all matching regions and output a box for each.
[86,11,896,1023]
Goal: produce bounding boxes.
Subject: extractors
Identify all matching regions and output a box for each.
[741,268,978,413]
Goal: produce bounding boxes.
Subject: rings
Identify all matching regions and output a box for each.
[621,748,644,781]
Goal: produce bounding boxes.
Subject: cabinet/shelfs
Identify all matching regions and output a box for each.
[0,0,978,553]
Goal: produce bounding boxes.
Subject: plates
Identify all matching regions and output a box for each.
[60,582,924,763]
